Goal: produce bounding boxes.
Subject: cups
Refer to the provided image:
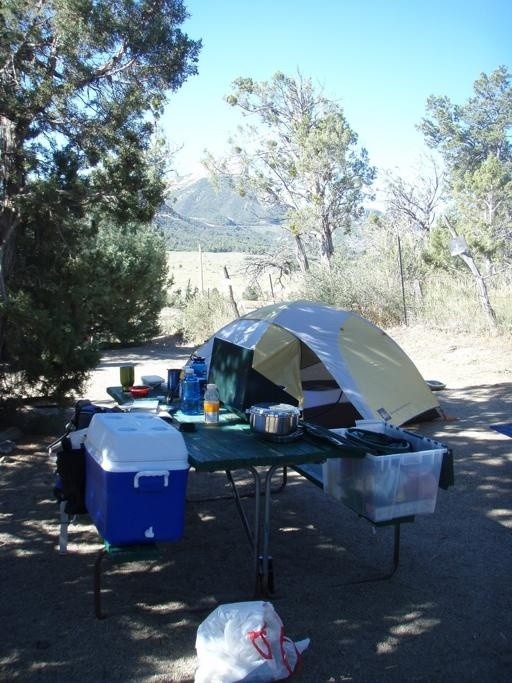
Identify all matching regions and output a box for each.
[120,366,134,394]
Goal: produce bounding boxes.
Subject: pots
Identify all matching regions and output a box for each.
[246,405,304,436]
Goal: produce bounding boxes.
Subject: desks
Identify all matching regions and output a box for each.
[107,382,366,599]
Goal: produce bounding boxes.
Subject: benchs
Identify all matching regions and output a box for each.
[281,460,416,575]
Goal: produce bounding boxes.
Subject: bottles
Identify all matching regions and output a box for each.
[189,353,209,407]
[180,368,200,416]
[204,384,220,426]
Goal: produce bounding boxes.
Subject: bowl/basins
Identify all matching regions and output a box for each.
[129,386,153,397]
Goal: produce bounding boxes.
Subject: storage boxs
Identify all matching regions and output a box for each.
[323,422,448,524]
[83,411,190,542]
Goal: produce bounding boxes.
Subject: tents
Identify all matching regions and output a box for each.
[178,300,447,428]
